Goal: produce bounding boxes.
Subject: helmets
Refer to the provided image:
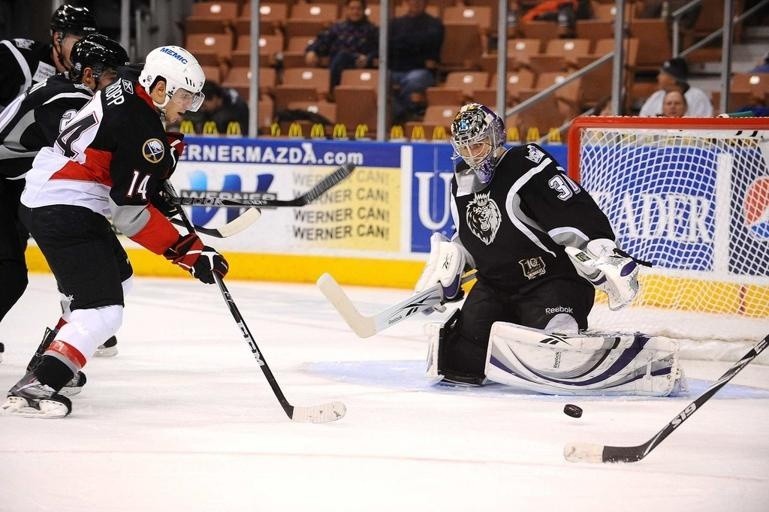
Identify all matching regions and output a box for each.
[69,34,131,78]
[138,45,205,112]
[48,4,100,39]
[450,103,506,185]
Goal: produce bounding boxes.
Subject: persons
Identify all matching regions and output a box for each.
[387,0,444,126]
[303,0,378,87]
[662,90,688,117]
[200,80,249,138]
[5,44,229,415]
[416,103,639,390]
[639,57,713,117]
[0,33,131,355]
[0,1,97,114]
[593,96,612,116]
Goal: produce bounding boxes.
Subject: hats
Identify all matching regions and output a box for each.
[661,58,689,81]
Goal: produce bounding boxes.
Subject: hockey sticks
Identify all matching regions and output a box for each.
[315,272,477,339]
[563,336,769,463]
[170,163,356,208]
[162,179,346,423]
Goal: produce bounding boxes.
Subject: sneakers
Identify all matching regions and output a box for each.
[6,334,117,417]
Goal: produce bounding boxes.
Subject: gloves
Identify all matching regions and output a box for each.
[164,233,228,284]
